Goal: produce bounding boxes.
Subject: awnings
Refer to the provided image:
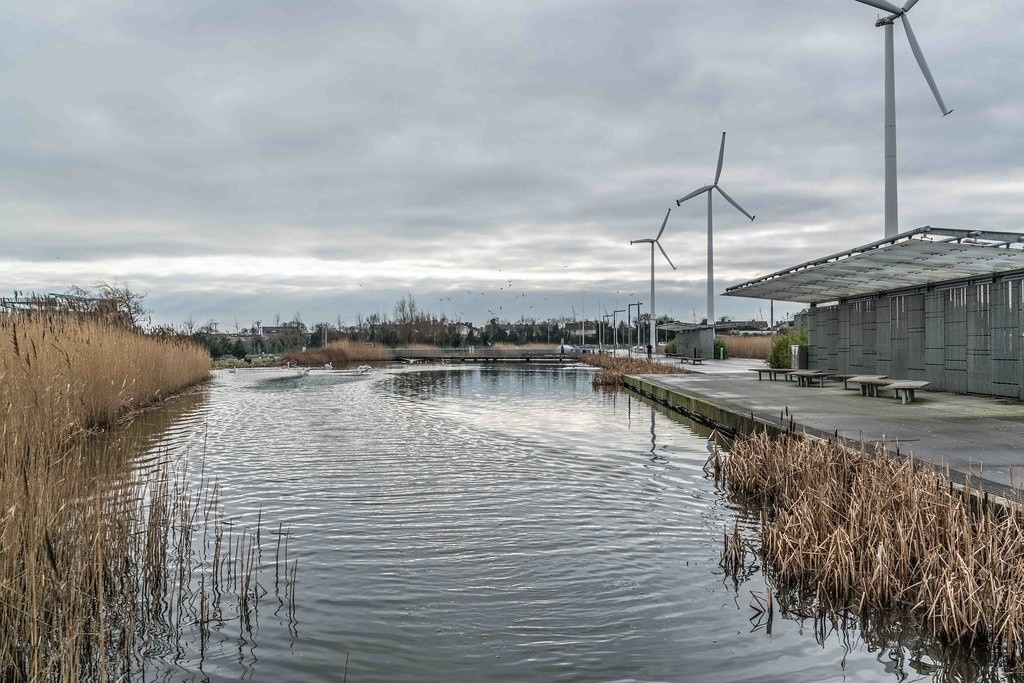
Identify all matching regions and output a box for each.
[656,322,713,331]
[719,226,1024,303]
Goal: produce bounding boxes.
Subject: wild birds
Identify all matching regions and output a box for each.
[358,265,634,323]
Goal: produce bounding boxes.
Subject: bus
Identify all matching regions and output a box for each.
[244,355,276,362]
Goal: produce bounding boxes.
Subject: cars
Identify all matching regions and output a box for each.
[658,342,668,346]
[635,344,643,350]
[220,355,237,360]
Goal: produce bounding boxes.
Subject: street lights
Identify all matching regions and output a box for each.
[614,310,626,359]
[629,293,640,349]
[603,314,614,354]
[547,318,550,344]
[209,322,218,340]
[616,291,620,349]
[627,303,643,348]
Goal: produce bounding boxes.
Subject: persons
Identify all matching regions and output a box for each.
[647,343,653,358]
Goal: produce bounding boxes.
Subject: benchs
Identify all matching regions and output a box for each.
[748,369,836,388]
[662,353,708,365]
[836,374,930,405]
